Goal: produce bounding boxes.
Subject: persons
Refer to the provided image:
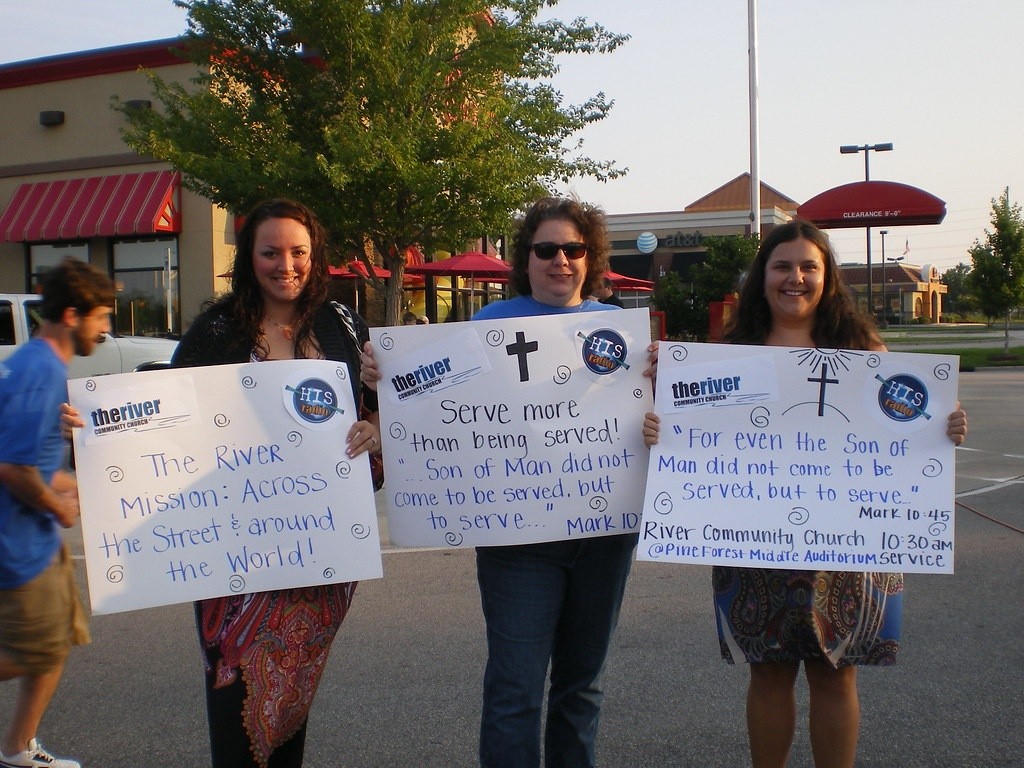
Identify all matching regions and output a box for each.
[586,276,624,307]
[401,311,430,325]
[644,218,968,768]
[357,193,660,768]
[1,256,121,768]
[57,197,380,766]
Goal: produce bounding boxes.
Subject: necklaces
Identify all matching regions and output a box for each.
[262,312,309,340]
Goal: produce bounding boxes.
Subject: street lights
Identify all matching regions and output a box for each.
[878,229,892,326]
[839,143,895,312]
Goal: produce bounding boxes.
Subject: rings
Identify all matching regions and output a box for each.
[371,438,378,444]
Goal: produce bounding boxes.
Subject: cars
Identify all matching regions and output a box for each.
[0,294,182,492]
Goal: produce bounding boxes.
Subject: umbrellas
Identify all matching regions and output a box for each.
[318,248,655,292]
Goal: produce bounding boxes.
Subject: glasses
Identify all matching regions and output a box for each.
[528,241,588,259]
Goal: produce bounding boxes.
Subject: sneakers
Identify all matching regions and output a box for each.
[1,738,81,768]
